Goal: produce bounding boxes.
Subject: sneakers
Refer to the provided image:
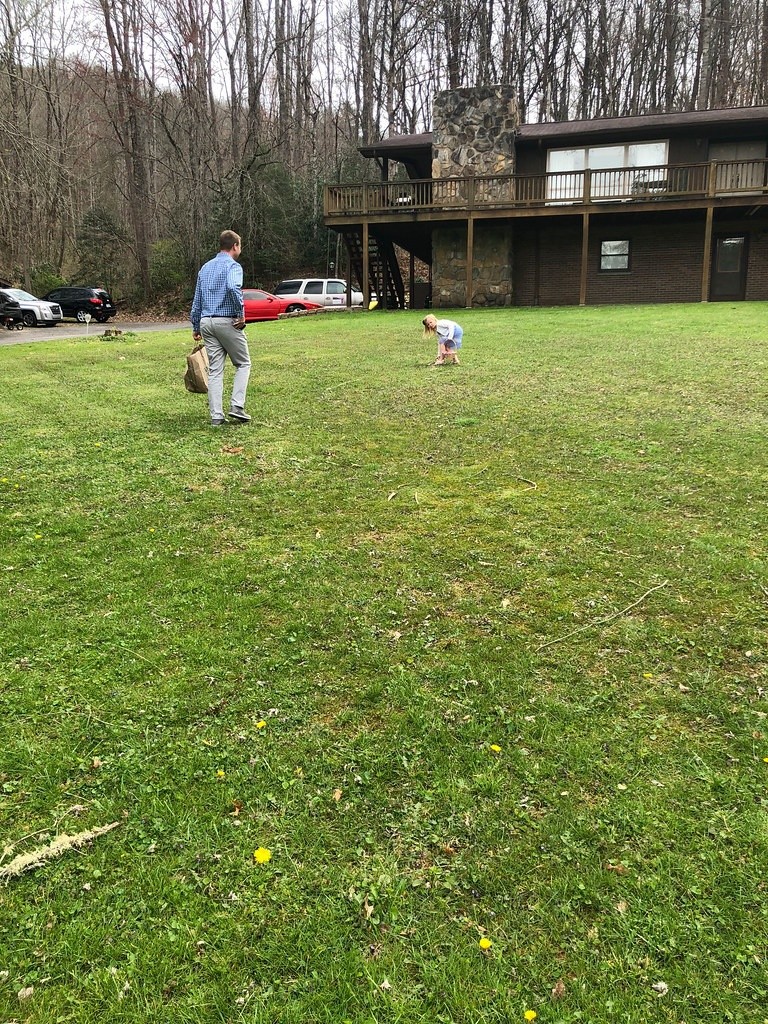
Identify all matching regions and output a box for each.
[211,419,229,426]
[228,405,251,420]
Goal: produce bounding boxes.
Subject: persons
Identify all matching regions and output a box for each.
[423,314,463,366]
[190,230,251,426]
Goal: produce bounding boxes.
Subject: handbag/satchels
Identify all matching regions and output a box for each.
[184,341,210,393]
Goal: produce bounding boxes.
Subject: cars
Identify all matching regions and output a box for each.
[241,288,323,322]
[0,288,63,328]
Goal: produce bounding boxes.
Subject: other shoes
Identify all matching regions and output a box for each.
[434,360,446,366]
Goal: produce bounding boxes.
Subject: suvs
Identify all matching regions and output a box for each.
[274,278,383,306]
[39,287,116,323]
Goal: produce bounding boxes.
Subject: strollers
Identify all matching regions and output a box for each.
[0,300,24,330]
